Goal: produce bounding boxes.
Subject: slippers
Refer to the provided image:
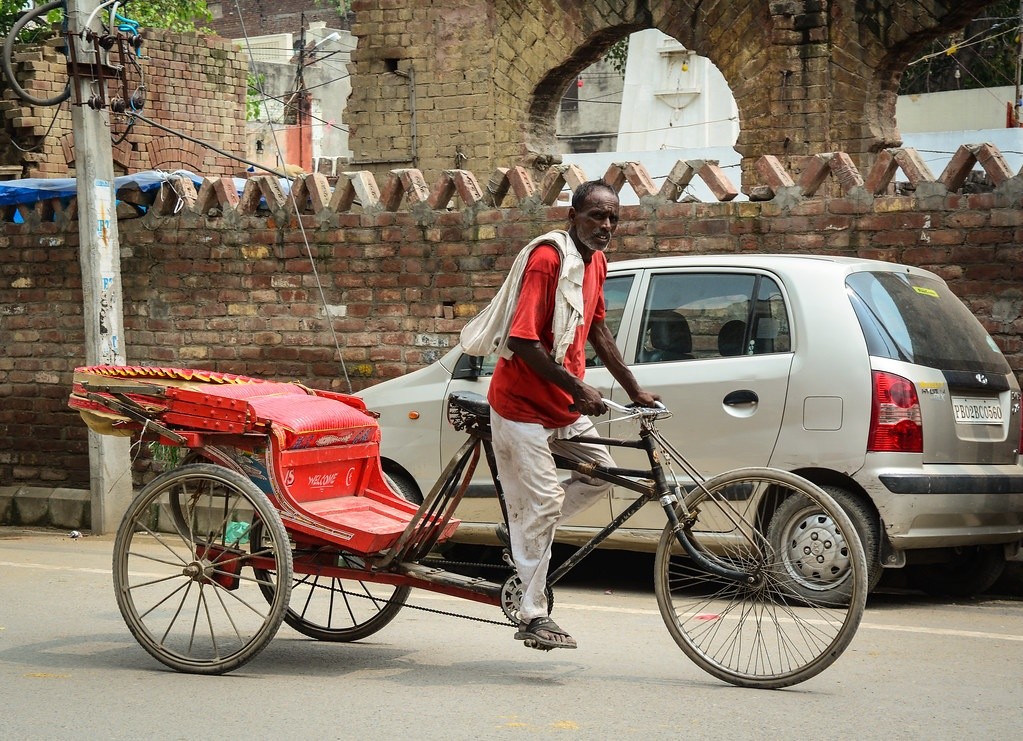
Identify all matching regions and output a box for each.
[513,616,577,649]
[496,522,512,551]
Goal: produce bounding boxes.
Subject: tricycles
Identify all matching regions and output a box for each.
[68,366,870,690]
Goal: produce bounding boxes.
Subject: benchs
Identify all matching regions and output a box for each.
[191,378,384,464]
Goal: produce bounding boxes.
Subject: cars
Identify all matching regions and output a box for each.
[341,251,1023,607]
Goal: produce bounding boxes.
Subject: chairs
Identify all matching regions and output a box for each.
[717,320,747,357]
[650,311,696,362]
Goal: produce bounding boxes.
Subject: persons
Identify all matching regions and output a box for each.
[487,180,662,649]
